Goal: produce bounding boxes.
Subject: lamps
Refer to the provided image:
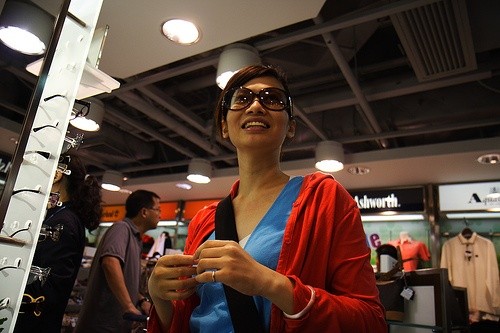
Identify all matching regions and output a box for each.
[69,96,105,131]
[0,0,54,55]
[215,42,262,90]
[100,168,123,191]
[314,139,345,172]
[187,158,212,183]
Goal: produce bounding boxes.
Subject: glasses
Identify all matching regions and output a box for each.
[222,87,293,120]
[147,208,162,214]
[0,94,91,317]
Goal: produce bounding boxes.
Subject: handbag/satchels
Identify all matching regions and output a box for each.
[373,261,405,322]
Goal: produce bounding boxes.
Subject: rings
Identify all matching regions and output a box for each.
[212,270,217,283]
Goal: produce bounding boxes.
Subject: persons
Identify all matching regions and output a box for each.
[146,63,389,332]
[72,186,161,332]
[12,144,106,332]
[386,231,431,270]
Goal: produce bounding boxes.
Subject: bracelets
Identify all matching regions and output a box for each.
[137,296,150,307]
[282,283,316,320]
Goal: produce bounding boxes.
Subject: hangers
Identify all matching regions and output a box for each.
[461,216,474,240]
